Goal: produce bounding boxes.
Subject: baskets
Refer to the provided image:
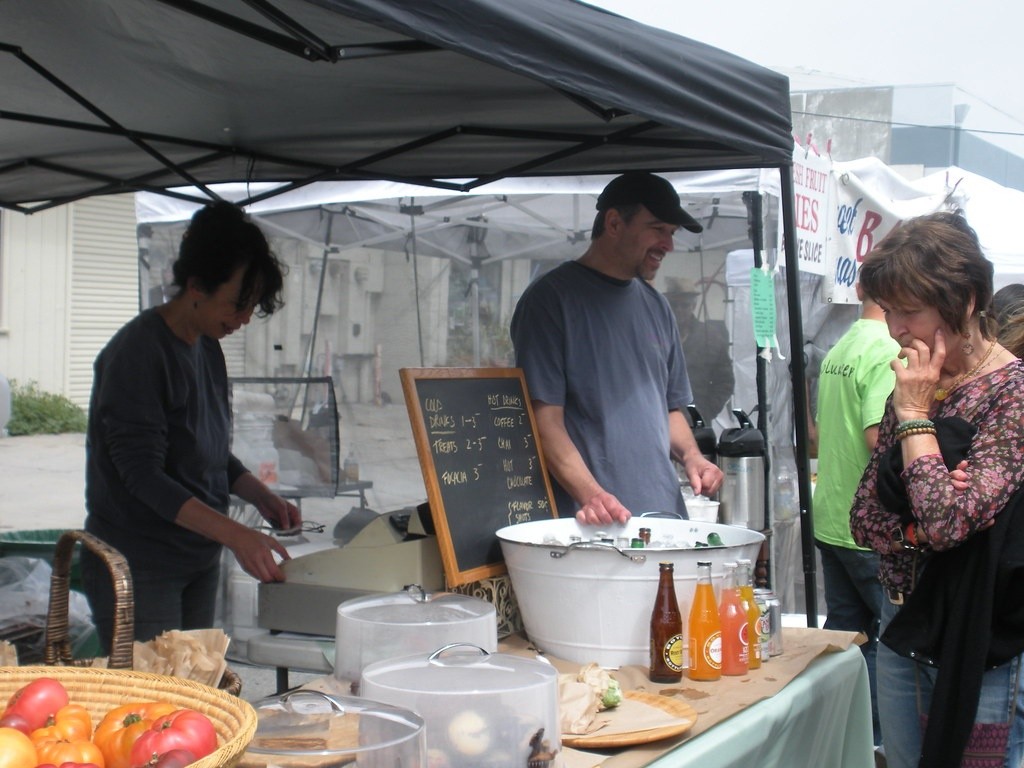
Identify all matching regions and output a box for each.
[0,666,260,768]
[20,531,243,697]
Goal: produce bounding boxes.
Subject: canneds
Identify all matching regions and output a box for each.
[752,588,783,662]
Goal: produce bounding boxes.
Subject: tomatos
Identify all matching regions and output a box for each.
[0,677,218,768]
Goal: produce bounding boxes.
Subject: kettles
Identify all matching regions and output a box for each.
[717,409,771,532]
[686,403,717,464]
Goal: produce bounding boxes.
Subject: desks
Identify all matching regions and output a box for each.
[251,632,335,693]
[229,478,375,536]
[499,634,877,768]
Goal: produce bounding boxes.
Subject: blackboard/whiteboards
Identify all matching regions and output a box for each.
[395,367,559,587]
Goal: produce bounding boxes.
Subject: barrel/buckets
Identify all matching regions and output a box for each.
[496,510,765,674]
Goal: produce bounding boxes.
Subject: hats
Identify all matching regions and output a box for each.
[598,171,704,233]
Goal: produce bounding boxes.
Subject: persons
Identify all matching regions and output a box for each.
[812,252,885,768]
[81,201,303,657]
[661,277,736,464]
[511,173,725,526]
[849,211,1024,768]
[986,283,1024,362]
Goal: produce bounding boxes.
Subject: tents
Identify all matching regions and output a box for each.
[915,166,1024,277]
[136,153,930,612]
[1,0,818,627]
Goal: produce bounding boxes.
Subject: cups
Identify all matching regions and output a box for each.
[685,501,719,522]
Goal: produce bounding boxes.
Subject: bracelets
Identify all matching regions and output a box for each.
[913,521,921,548]
[895,418,937,443]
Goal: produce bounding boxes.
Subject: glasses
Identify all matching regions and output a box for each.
[251,520,327,538]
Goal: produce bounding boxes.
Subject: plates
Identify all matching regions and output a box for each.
[562,689,698,747]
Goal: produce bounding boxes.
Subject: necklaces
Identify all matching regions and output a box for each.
[933,335,997,401]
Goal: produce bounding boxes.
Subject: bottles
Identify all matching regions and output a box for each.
[649,561,683,683]
[686,560,722,678]
[532,528,726,549]
[735,560,761,669]
[719,563,750,676]
